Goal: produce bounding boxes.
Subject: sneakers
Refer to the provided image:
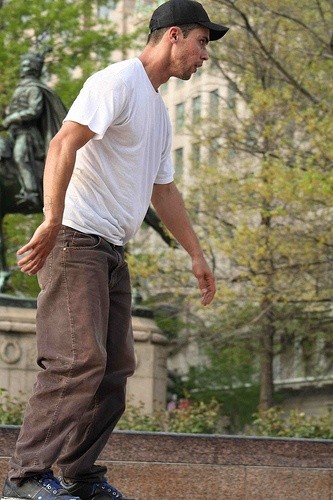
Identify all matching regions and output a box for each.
[1,473,81,500]
[57,475,135,500]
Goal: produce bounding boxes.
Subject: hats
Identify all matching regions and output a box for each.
[149,0,229,41]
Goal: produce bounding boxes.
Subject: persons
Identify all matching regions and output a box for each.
[167,393,191,419]
[2,54,70,212]
[1,1,229,500]
